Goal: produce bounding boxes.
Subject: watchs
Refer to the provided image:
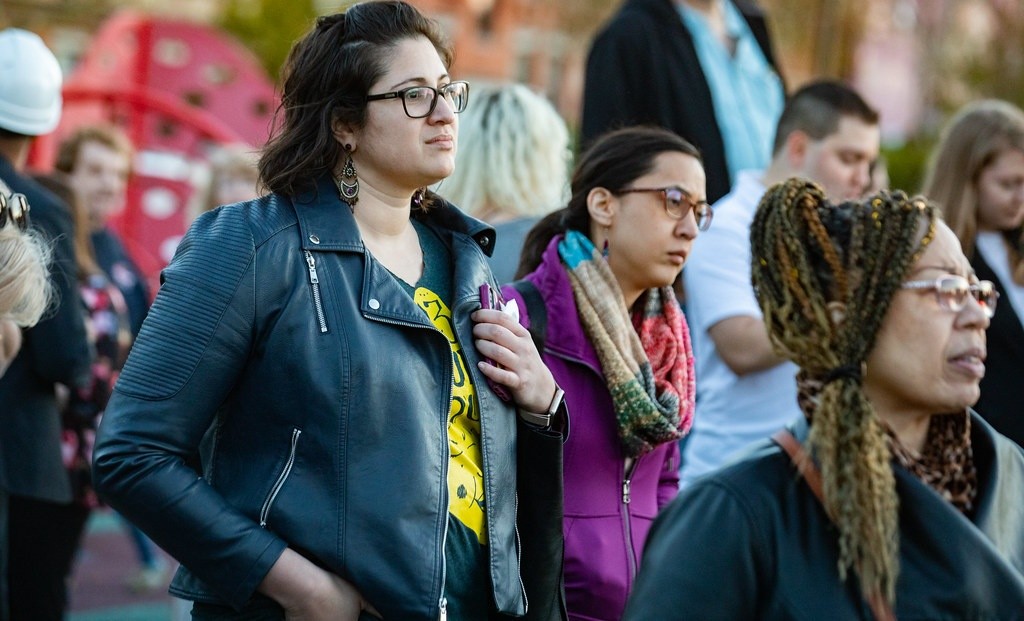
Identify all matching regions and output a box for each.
[519,380,565,427]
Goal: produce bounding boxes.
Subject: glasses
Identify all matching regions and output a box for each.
[617,185,714,232]
[366,79,470,119]
[901,274,1001,319]
[0,191,31,235]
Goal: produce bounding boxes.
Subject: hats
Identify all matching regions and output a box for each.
[0,27,64,136]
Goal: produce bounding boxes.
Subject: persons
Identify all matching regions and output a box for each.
[576,0,1024,493]
[426,75,578,289]
[92,0,572,621]
[624,177,1024,621]
[0,28,273,621]
[498,126,714,621]
